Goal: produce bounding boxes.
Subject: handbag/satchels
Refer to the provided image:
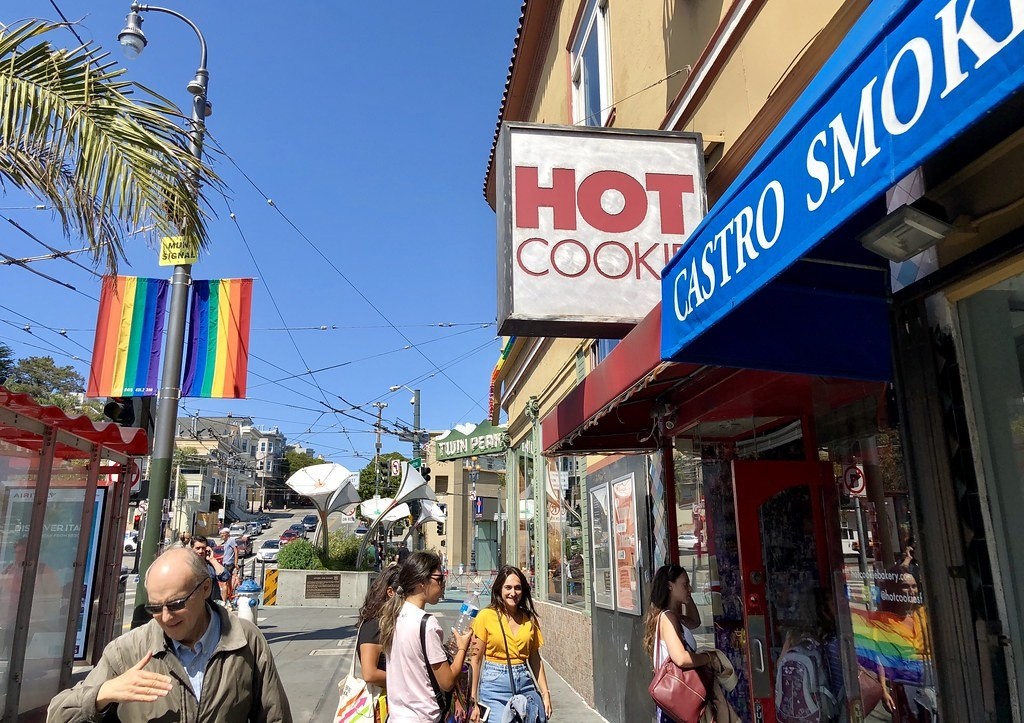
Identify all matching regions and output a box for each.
[331,617,384,723]
[232,568,241,592]
[857,663,884,719]
[510,691,547,723]
[914,687,938,723]
[648,610,714,723]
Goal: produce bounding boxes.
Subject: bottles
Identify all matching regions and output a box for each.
[570,581,576,596]
[446,592,481,655]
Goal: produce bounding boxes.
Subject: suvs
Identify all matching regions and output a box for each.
[256,515,271,529]
[228,522,253,538]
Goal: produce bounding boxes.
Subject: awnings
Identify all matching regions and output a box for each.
[660,0,1024,382]
[540,304,890,457]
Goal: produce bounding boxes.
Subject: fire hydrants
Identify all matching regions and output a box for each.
[233,579,262,626]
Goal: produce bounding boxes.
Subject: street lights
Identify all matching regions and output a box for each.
[390,384,421,554]
[115,0,212,633]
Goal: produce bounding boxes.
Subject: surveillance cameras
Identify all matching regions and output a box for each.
[410,399,415,405]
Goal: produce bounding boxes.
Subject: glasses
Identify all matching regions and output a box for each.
[429,572,444,585]
[144,576,210,614]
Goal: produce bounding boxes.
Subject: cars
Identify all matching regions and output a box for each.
[212,546,224,566]
[355,528,374,542]
[251,521,262,536]
[236,537,254,559]
[279,530,303,545]
[289,524,307,540]
[301,515,319,532]
[206,539,217,548]
[123,532,172,555]
[256,539,285,563]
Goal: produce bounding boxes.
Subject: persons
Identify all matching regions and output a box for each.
[867,547,939,723]
[356,547,473,723]
[775,589,838,723]
[267,500,272,511]
[45,546,293,723]
[0,538,56,693]
[469,566,553,723]
[642,563,721,723]
[258,505,263,513]
[171,519,239,602]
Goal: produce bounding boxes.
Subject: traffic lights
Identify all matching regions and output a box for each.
[437,521,444,536]
[134,515,140,529]
[421,467,431,481]
[103,394,150,432]
[441,540,445,547]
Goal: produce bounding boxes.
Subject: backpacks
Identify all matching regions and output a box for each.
[775,633,847,723]
[420,613,475,723]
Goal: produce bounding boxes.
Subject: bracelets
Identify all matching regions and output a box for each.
[456,649,466,653]
[700,650,711,662]
[541,690,551,695]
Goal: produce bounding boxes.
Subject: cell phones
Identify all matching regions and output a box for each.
[205,550,212,558]
[477,702,491,722]
